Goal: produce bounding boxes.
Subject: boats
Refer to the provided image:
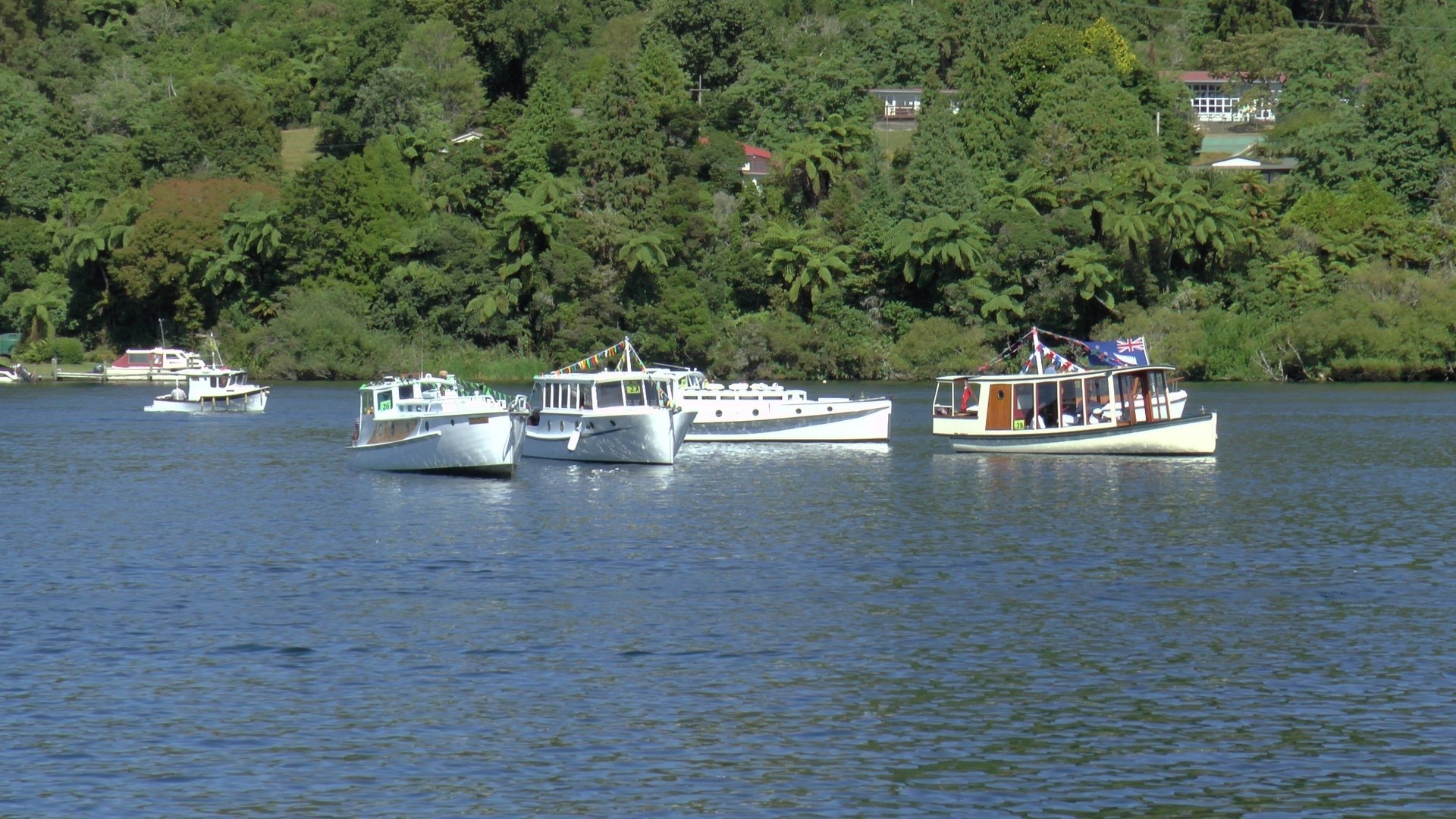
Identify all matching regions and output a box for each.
[640,363,889,445]
[511,336,696,467]
[143,367,271,414]
[0,352,39,384]
[345,334,529,480]
[930,328,1219,457]
[102,347,210,383]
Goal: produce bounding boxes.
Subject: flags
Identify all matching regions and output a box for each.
[960,381,971,411]
[1082,336,1149,368]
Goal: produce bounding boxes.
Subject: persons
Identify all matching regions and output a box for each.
[1044,361,1056,374]
[1036,382,1060,427]
[1075,388,1103,417]
[169,383,188,401]
